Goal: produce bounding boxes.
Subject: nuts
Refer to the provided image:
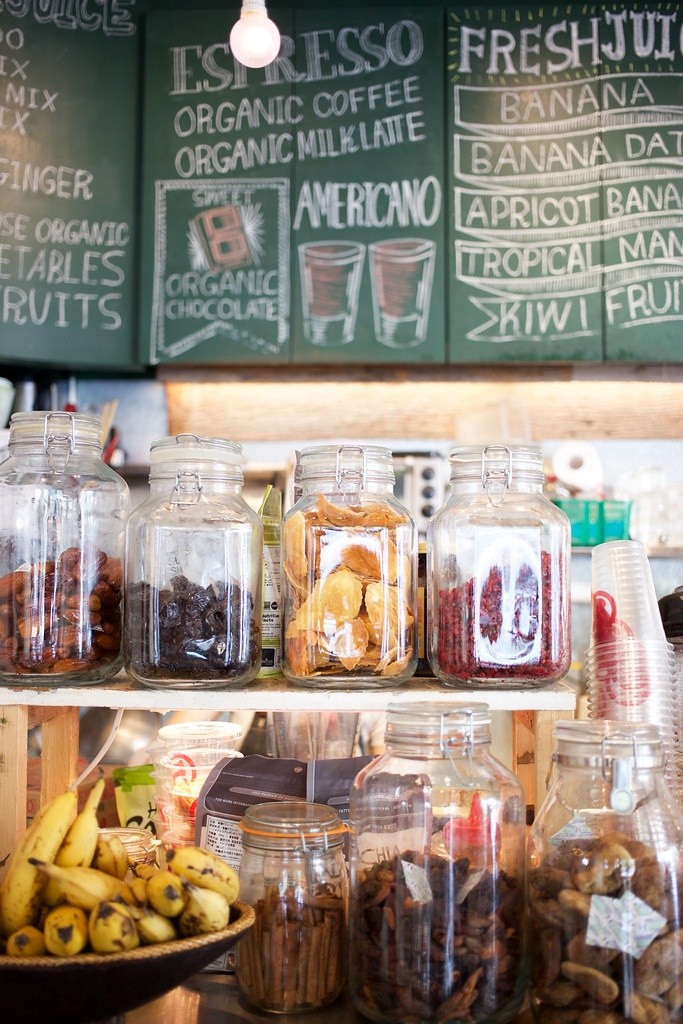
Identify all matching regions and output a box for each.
[0,547,126,675]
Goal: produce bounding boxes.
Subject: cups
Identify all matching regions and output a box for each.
[585,539,683,835]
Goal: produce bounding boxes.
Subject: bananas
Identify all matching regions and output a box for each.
[0,776,241,957]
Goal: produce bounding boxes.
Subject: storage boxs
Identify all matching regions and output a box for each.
[556,502,635,547]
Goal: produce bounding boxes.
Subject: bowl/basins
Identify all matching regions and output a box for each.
[0,898,257,1024]
[151,723,243,845]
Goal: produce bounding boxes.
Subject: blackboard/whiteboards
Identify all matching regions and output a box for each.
[0,1,152,370]
[138,0,683,367]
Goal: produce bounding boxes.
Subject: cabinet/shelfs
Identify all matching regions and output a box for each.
[105,456,683,653]
[0,676,582,1024]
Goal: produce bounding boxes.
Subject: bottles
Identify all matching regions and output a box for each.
[121,434,265,689]
[281,444,419,690]
[529,719,683,1024]
[238,801,350,1014]
[347,699,526,1024]
[427,446,572,689]
[87,823,159,867]
[0,410,132,688]
